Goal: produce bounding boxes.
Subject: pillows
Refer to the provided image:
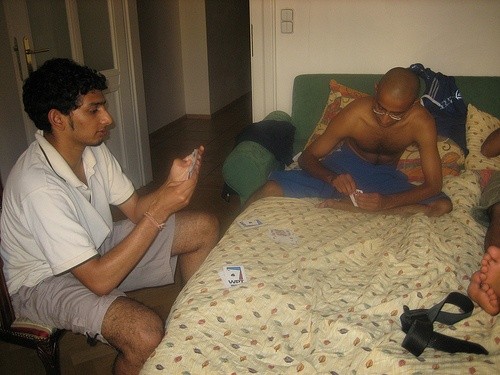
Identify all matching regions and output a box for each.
[302,79,465,182]
[466,103,500,169]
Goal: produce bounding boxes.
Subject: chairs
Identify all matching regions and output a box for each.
[0,270,68,375]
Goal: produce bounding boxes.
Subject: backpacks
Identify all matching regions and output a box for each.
[409,63,467,123]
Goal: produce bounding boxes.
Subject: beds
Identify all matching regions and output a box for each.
[138,74,500,375]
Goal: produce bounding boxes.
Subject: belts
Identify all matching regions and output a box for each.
[400,292,489,357]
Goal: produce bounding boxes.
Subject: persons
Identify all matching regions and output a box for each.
[1,60,221,373]
[467,128,499,316]
[244,67,455,216]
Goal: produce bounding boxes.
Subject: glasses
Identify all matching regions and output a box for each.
[372,97,417,121]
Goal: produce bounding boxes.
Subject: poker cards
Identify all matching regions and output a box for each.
[219,265,246,289]
[186,148,200,179]
[239,218,263,228]
[269,227,298,245]
[348,189,364,208]
